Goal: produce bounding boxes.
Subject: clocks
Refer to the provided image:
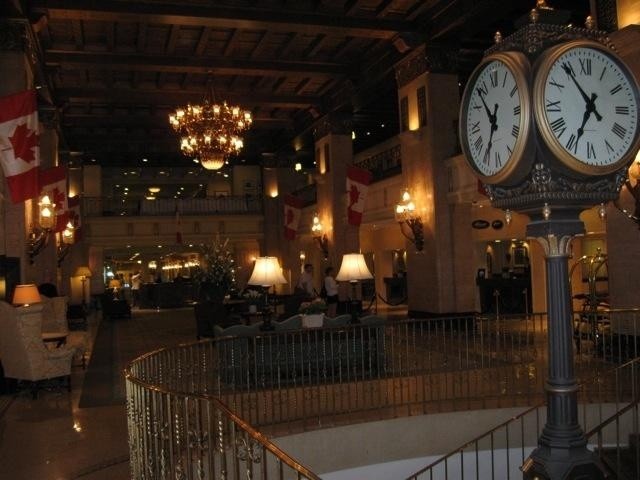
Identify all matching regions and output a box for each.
[459,51,536,190]
[530,40,640,178]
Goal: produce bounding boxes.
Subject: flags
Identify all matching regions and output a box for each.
[175,208,184,245]
[282,192,304,242]
[346,164,373,227]
[0,88,83,245]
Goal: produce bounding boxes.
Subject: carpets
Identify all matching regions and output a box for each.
[77,310,512,409]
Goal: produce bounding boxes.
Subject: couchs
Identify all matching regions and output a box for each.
[133,282,204,308]
[263,294,309,321]
[42,296,68,351]
[214,314,386,392]
[0,301,73,399]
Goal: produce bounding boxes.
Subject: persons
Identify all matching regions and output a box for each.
[295,264,317,307]
[521,257,531,274]
[106,267,184,309]
[324,266,344,319]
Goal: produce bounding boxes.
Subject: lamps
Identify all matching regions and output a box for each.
[76,266,92,325]
[33,194,52,260]
[12,283,42,307]
[168,72,254,171]
[108,279,121,300]
[247,256,288,332]
[311,216,328,259]
[58,223,73,264]
[336,253,373,315]
[395,189,424,252]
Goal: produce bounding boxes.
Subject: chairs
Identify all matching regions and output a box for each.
[101,292,132,318]
[194,302,240,339]
